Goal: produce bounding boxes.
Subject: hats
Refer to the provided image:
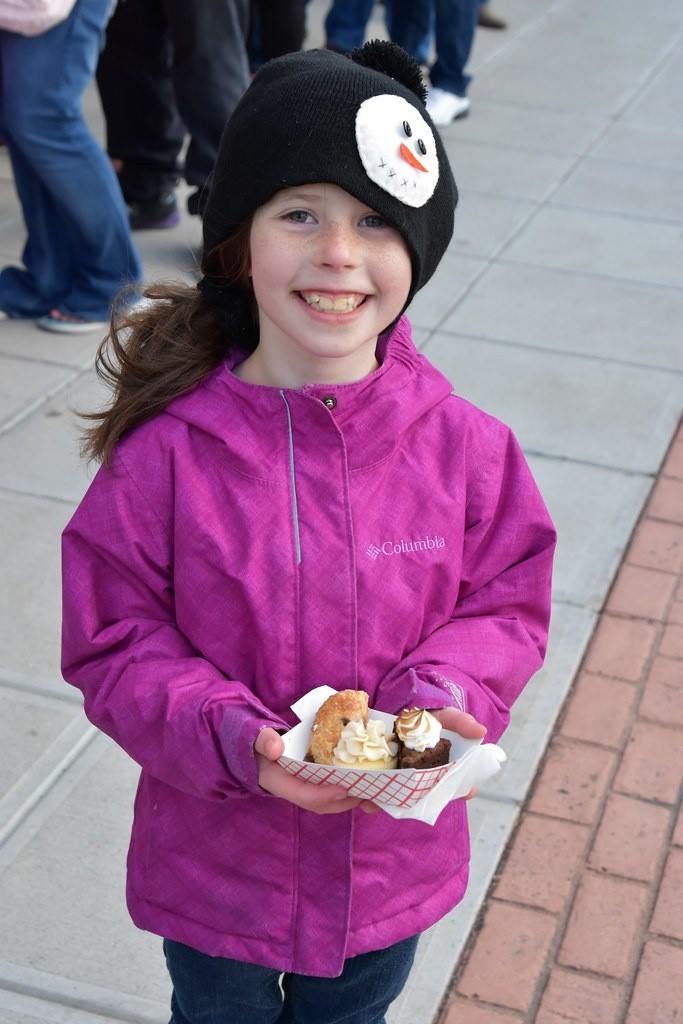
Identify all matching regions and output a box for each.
[203,39,458,335]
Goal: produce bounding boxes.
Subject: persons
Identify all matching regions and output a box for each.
[61,47,555,1024]
[1,0,509,338]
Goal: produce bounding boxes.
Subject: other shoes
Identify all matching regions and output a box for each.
[39,307,113,332]
[426,86,471,126]
[129,198,178,229]
[478,6,508,29]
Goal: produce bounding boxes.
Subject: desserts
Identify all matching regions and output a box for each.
[392,706,452,770]
[309,688,400,771]
